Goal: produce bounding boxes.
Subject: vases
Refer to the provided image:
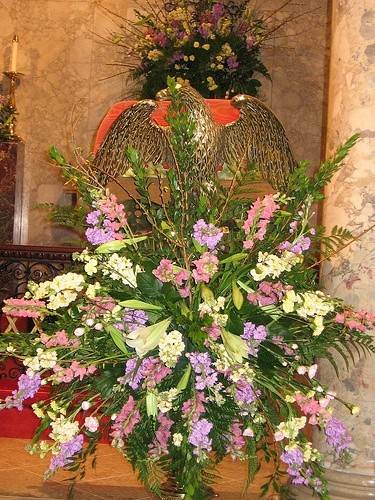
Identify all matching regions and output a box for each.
[94,98,240,161]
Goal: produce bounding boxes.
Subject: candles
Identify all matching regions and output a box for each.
[11,42,18,72]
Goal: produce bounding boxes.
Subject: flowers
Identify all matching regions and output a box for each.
[87,0,322,102]
[0,74,375,500]
